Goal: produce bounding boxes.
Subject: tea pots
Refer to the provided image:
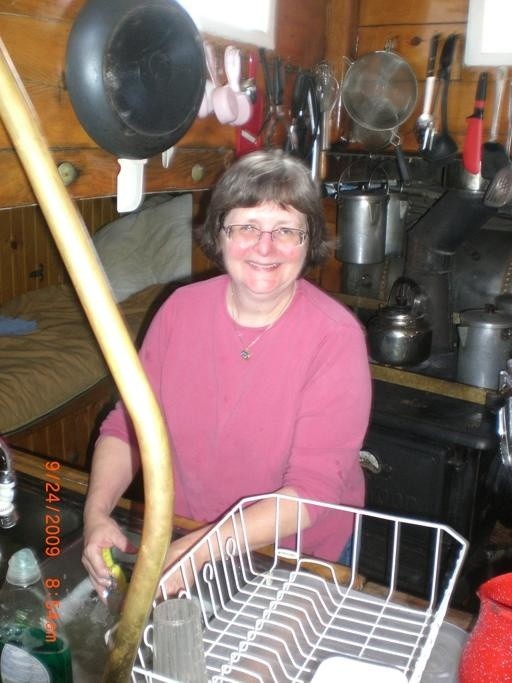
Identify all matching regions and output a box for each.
[340,189,409,266]
[366,277,434,367]
[454,304,512,390]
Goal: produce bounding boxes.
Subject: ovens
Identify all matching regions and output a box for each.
[356,412,498,597]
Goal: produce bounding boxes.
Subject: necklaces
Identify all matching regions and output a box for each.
[227,280,299,359]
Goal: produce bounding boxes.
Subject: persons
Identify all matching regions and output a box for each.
[81,149,373,601]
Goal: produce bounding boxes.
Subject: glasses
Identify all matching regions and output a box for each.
[220,224,310,246]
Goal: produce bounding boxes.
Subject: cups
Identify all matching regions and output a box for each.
[150,599,209,683]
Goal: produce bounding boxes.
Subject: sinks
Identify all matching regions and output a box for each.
[0,470,84,581]
[40,513,313,683]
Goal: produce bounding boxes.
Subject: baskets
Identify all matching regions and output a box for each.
[106,495,470,683]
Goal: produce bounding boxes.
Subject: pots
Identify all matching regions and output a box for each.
[65,0,208,155]
